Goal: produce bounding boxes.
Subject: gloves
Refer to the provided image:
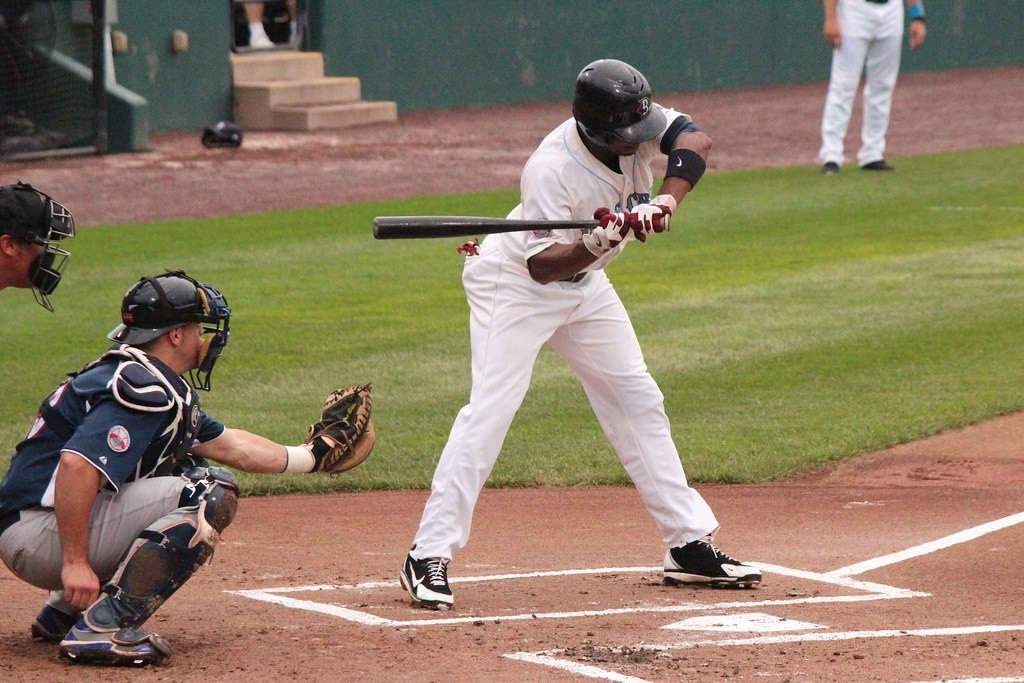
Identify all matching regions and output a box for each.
[632,195,677,243]
[583,208,631,258]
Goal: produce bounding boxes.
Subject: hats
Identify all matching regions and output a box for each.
[0,181,72,240]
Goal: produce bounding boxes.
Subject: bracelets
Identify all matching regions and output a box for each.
[663,146,707,193]
[908,3,926,22]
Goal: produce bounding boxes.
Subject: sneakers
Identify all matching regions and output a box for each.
[398,549,454,611]
[663,542,764,590]
[32,604,77,642]
[59,621,163,666]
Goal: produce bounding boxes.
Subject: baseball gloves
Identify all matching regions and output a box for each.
[305,379,379,480]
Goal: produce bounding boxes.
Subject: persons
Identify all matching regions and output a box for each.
[0,269,376,666]
[400,58,763,609]
[817,0,927,176]
[0,182,75,312]
[244,0,304,50]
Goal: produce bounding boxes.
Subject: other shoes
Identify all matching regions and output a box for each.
[861,160,895,172]
[821,163,840,177]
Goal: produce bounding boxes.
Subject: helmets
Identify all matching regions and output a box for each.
[105,274,205,345]
[201,119,243,148]
[573,58,668,147]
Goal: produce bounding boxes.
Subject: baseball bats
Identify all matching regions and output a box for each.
[372,213,672,241]
